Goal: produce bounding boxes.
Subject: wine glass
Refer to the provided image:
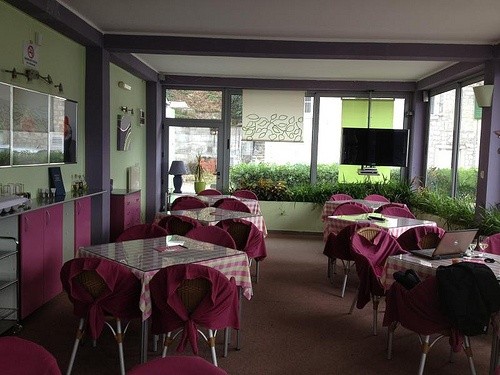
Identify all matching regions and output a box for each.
[479,235,489,258]
[468,238,477,257]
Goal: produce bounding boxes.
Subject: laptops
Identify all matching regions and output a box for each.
[410,228,479,260]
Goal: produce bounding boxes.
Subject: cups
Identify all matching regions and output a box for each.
[0,182,24,195]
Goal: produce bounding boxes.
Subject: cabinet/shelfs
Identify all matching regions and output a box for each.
[74,197,90,258]
[43,204,63,305]
[20,208,44,321]
[110,190,141,243]
[0,215,22,337]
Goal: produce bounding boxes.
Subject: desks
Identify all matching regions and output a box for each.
[193,194,262,215]
[380,248,500,375]
[323,212,437,292]
[153,207,269,283]
[320,199,412,274]
[78,235,254,365]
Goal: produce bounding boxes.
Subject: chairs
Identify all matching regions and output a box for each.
[0,189,500,375]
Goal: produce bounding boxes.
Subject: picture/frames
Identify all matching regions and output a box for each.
[0,81,79,169]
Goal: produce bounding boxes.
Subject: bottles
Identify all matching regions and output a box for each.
[72,174,87,190]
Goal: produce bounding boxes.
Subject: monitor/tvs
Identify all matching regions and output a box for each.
[340,127,409,168]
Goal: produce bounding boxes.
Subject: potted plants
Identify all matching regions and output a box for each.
[193,150,206,194]
[229,172,500,251]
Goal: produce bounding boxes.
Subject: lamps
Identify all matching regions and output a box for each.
[54,83,66,95]
[11,66,20,83]
[128,108,135,116]
[25,69,35,86]
[44,74,56,89]
[118,81,132,91]
[121,106,129,115]
[168,161,188,194]
[473,85,494,107]
